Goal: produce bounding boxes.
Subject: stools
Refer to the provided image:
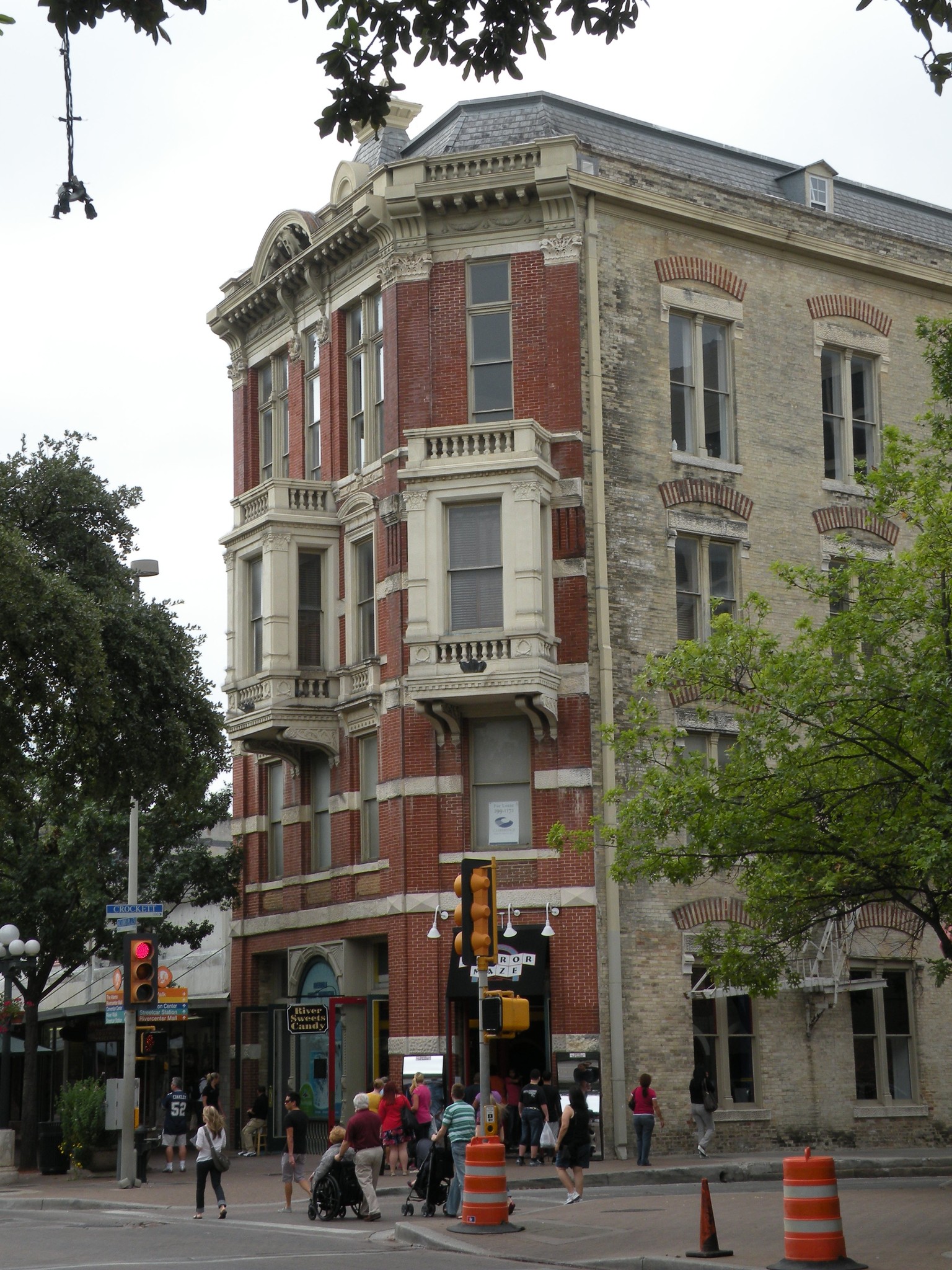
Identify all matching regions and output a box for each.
[253,1125,268,1156]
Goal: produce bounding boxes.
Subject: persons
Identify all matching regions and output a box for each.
[366,1076,449,1188]
[462,1063,525,1152]
[473,1092,515,1216]
[187,1063,227,1129]
[409,1073,431,1173]
[238,1086,268,1156]
[517,1071,557,1166]
[628,1073,665,1166]
[573,1061,600,1101]
[430,1084,476,1218]
[689,1067,718,1158]
[554,1086,590,1205]
[378,1082,411,1176]
[536,1072,562,1164]
[192,1105,230,1219]
[333,1093,384,1222]
[162,1077,189,1172]
[307,1126,356,1205]
[277,1093,313,1213]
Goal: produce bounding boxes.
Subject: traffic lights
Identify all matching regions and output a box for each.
[123,933,158,1011]
[481,995,530,1036]
[140,1030,168,1057]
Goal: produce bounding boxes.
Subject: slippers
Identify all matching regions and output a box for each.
[193,1214,203,1219]
[218,1209,227,1219]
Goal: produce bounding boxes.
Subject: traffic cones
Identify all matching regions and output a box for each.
[684,1178,733,1258]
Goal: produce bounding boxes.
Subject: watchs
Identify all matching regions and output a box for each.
[338,1152,344,1158]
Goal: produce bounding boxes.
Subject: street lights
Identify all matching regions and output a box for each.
[0,923,39,1187]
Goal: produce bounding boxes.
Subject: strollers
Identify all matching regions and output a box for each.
[401,1134,455,1218]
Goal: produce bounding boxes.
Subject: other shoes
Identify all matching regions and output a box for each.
[573,1198,583,1203]
[162,1167,173,1173]
[357,1215,369,1219]
[564,1191,580,1205]
[516,1158,526,1166]
[529,1161,539,1166]
[409,1169,419,1173]
[243,1152,256,1157]
[278,1206,292,1213]
[697,1145,708,1156]
[238,1151,247,1155]
[508,1202,515,1215]
[537,1159,545,1164]
[457,1215,463,1219]
[363,1213,381,1221]
[402,1172,408,1176]
[407,1181,413,1188]
[699,1155,708,1159]
[552,1160,557,1164]
[379,1173,384,1175]
[637,1162,652,1166]
[385,1164,390,1169]
[389,1172,395,1176]
[180,1166,187,1172]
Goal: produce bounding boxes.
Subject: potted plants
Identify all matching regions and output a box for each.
[54,1076,118,1172]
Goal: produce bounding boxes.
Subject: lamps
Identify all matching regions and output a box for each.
[541,902,559,936]
[503,903,520,937]
[427,905,449,938]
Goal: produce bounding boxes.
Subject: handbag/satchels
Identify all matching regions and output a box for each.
[499,1125,505,1143]
[210,1145,231,1172]
[192,1100,204,1112]
[628,1094,636,1111]
[703,1089,718,1112]
[539,1121,556,1149]
[400,1106,420,1129]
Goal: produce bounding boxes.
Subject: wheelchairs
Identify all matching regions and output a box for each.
[308,1151,368,1222]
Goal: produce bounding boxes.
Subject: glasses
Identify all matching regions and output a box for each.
[284,1100,290,1103]
